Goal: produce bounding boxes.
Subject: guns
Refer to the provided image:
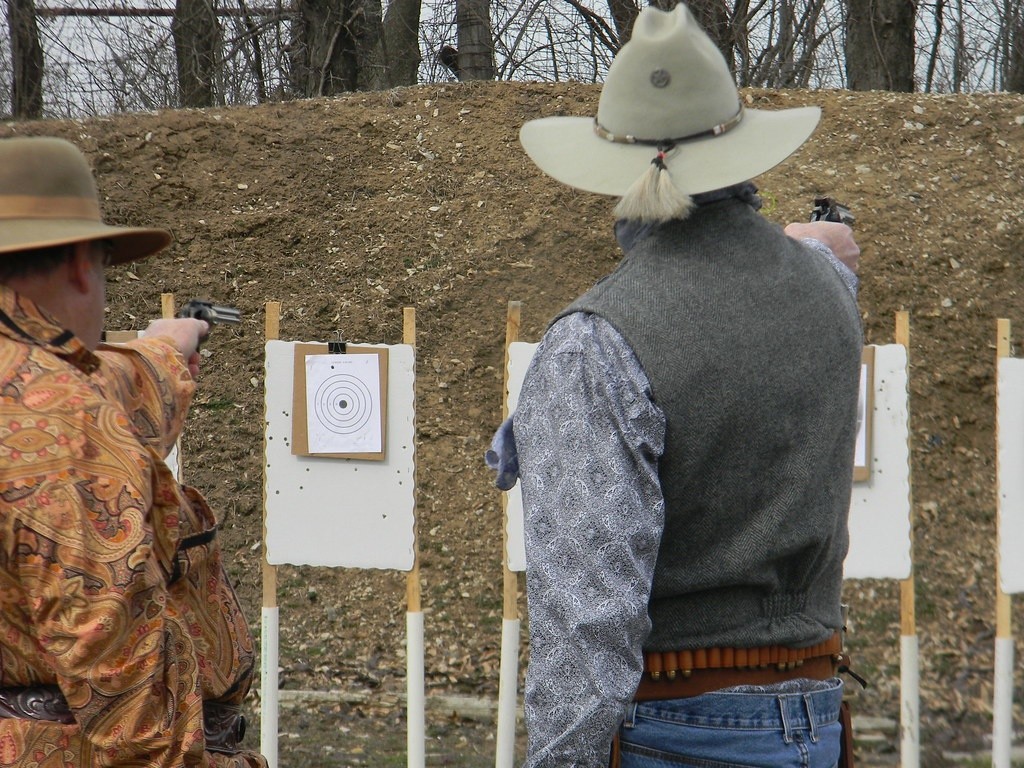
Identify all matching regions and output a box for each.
[177,298,243,344]
[809,198,856,228]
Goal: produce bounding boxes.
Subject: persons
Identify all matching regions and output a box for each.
[512,2,861,768]
[0,137,268,768]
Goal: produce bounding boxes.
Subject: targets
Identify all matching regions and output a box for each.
[314,373,373,435]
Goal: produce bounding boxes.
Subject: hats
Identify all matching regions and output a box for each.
[519,3,822,221]
[0,136,169,265]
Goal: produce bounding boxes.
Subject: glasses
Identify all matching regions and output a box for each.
[91,238,114,267]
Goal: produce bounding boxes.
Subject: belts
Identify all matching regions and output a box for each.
[0,686,246,754]
[634,654,834,704]
